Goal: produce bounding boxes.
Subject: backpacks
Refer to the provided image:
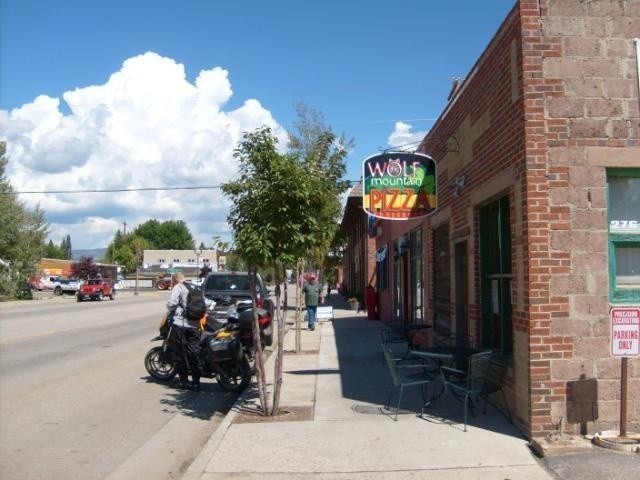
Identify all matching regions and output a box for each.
[178,281,205,321]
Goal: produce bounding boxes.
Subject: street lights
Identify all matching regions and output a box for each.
[194,248,202,282]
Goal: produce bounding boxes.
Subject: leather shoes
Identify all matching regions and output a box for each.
[170,379,189,388]
[186,381,201,392]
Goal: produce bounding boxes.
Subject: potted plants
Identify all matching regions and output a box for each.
[347,297,361,312]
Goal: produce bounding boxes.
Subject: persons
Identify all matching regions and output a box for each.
[163,271,207,393]
[300,274,325,331]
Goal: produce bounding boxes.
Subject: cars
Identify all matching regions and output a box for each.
[298,272,319,287]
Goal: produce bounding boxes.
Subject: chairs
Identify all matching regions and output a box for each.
[377,316,514,432]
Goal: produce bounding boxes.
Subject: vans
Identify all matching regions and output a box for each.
[198,270,268,322]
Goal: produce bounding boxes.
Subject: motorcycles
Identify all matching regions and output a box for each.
[142,294,274,395]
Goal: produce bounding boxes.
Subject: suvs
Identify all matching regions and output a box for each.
[28,275,116,302]
